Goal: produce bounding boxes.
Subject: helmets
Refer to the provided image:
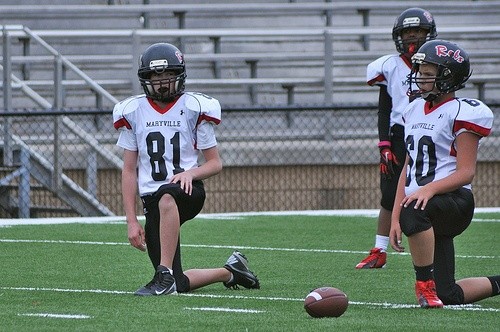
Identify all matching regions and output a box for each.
[392,8,438,53]
[136,42,187,100]
[406,40,474,108]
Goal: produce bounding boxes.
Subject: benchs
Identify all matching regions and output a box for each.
[0,0,500,145]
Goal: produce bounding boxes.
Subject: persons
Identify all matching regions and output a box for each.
[113,43,260,295]
[388,39,500,308]
[355,8,439,271]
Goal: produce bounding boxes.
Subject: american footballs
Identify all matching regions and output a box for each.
[303,286,349,318]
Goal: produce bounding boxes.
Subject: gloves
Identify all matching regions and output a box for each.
[378,140,403,181]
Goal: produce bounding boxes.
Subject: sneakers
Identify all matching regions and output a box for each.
[222,251,261,289]
[355,247,388,269]
[414,280,444,308]
[134,265,179,298]
